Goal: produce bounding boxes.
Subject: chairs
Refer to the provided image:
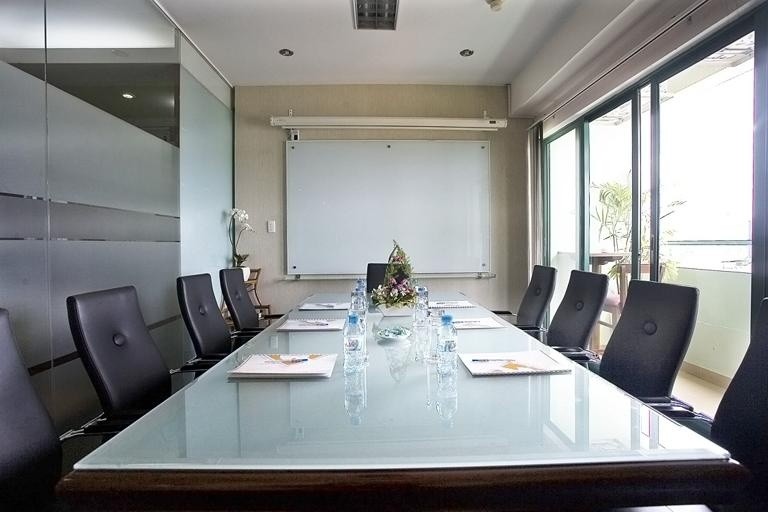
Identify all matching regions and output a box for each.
[218,269,288,353]
[65,283,217,445]
[591,262,664,354]
[658,295,768,481]
[492,265,557,341]
[176,273,262,380]
[564,278,702,410]
[516,270,614,357]
[0,309,125,510]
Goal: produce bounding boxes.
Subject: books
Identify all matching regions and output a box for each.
[457,348,572,377]
[223,351,339,378]
[449,317,508,330]
[298,302,352,311]
[427,299,477,309]
[276,318,346,331]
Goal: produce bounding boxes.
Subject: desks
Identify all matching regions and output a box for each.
[61,291,750,511]
[555,251,623,301]
[220,267,263,319]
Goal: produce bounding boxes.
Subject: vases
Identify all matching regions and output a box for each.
[241,267,250,282]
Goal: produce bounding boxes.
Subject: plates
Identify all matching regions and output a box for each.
[376,328,412,342]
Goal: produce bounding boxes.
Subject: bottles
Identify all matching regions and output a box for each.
[342,278,369,373]
[428,368,458,427]
[435,314,459,378]
[346,375,368,425]
[412,278,457,365]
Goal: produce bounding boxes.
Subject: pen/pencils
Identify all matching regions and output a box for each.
[436,301,459,304]
[316,303,333,308]
[472,358,516,362]
[299,323,328,326]
[265,358,308,363]
[453,320,481,323]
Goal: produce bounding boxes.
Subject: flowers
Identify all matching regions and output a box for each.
[226,209,255,268]
[370,241,418,308]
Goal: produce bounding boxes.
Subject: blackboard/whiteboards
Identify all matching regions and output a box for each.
[285,140,496,280]
